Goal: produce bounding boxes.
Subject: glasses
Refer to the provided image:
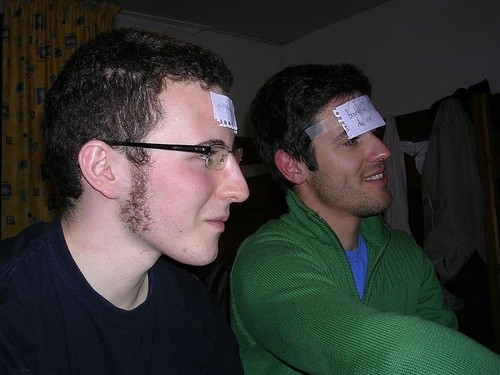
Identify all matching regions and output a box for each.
[102,141,243,170]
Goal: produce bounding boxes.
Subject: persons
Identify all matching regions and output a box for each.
[224,62,500,375]
[1,26,250,375]
